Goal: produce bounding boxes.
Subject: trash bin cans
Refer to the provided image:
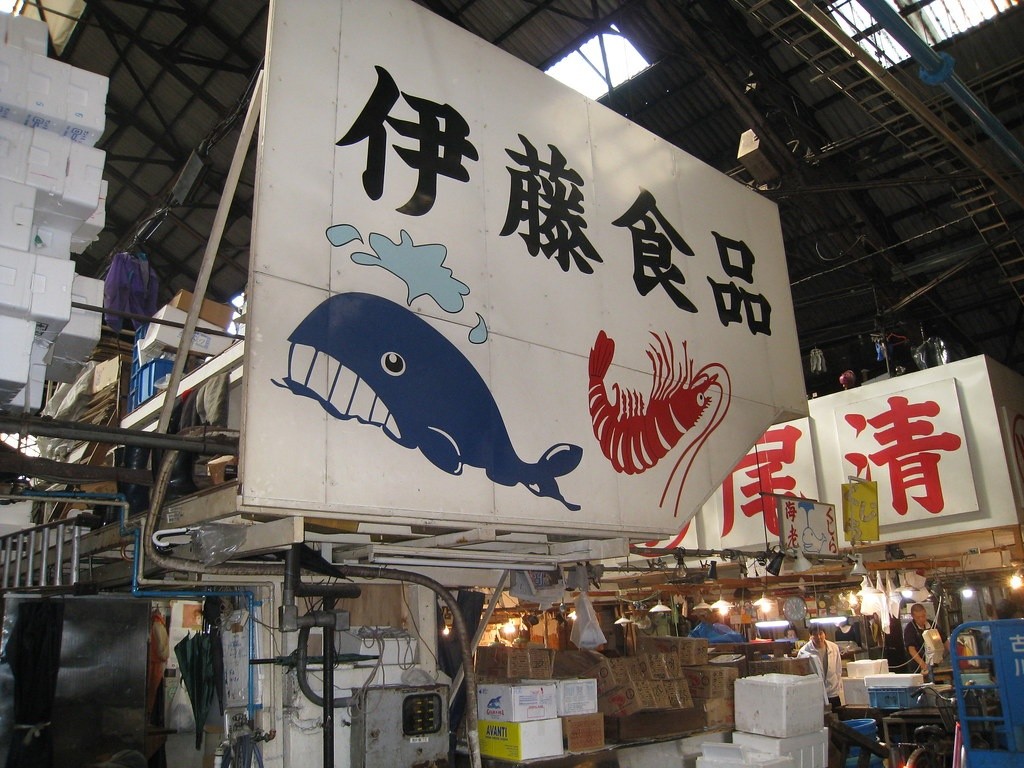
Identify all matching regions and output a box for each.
[841,718,878,756]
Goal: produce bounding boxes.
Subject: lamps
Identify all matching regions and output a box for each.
[613,606,634,626]
[894,573,921,598]
[710,587,736,614]
[567,611,576,619]
[955,580,978,598]
[785,546,812,572]
[648,594,672,612]
[847,552,868,576]
[765,554,785,577]
[692,596,711,610]
[855,576,884,597]
[753,590,775,610]
[706,560,718,581]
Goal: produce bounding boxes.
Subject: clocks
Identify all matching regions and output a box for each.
[783,596,807,621]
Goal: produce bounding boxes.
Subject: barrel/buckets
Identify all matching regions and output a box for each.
[843,719,884,768]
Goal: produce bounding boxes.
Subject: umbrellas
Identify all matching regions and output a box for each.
[173,620,215,751]
[203,595,225,715]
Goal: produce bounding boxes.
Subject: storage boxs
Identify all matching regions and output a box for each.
[0,10,110,417]
[732,727,828,768]
[842,659,923,705]
[127,288,234,413]
[696,741,794,768]
[734,673,824,738]
[334,625,418,667]
[455,635,809,761]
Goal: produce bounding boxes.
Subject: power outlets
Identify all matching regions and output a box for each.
[967,547,980,555]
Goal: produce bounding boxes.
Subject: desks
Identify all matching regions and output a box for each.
[882,704,999,768]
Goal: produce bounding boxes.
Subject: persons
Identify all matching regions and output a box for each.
[784,627,798,639]
[797,623,846,713]
[834,615,862,648]
[986,599,1017,684]
[904,604,950,683]
